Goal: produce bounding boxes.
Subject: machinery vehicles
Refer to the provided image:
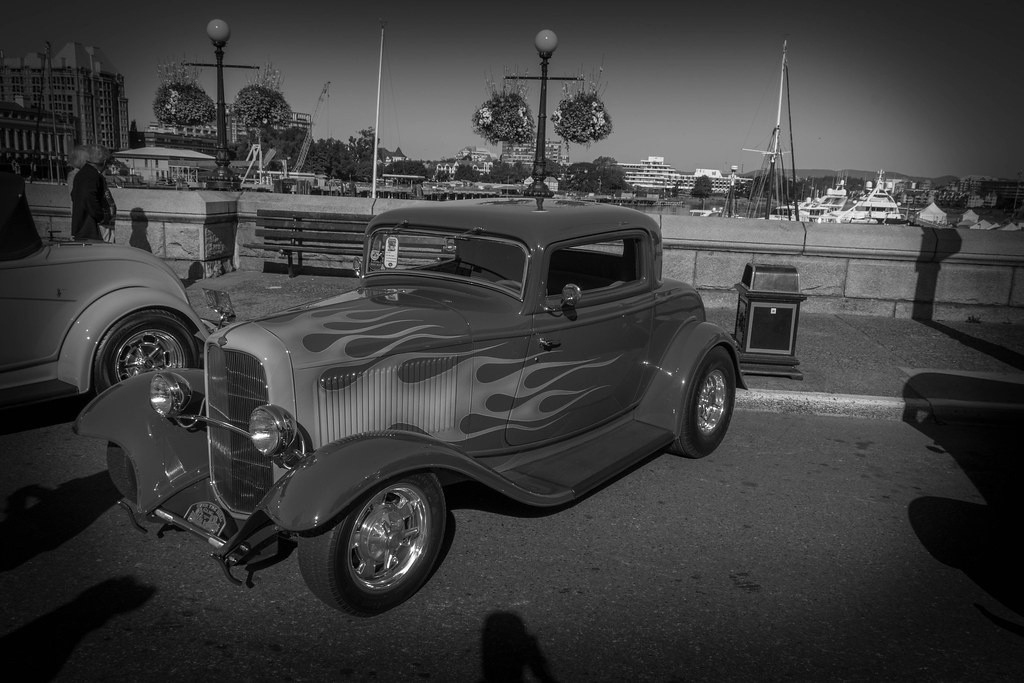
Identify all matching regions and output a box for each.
[289,81,332,173]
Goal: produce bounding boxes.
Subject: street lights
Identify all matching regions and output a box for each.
[179,17,261,192]
[502,30,587,198]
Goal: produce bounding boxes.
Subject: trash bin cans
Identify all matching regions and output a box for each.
[730,262,808,382]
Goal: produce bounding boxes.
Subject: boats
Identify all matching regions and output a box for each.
[686,34,1023,231]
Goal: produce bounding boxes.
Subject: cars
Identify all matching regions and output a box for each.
[67,195,749,616]
[0,148,209,419]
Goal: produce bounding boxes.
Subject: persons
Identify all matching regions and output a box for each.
[66,145,93,202]
[71,144,117,244]
